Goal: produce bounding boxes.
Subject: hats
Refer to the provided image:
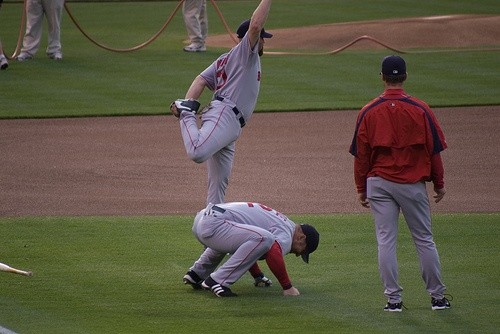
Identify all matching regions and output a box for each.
[381,55,406,75]
[301,224,320,264]
[236,19,273,39]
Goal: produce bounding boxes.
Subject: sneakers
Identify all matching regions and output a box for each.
[183,42,206,52]
[384,301,403,312]
[15,52,32,61]
[0,54,8,70]
[201,277,237,298]
[183,270,206,291]
[54,54,63,61]
[169,99,201,119]
[432,295,450,311]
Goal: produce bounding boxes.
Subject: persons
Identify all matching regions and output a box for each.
[15,0,64,61]
[184,201,320,299]
[348,55,454,311]
[183,0,207,51]
[0,42,9,69]
[170,0,273,255]
[0,263,33,277]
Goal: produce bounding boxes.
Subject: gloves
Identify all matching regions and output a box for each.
[254,273,273,289]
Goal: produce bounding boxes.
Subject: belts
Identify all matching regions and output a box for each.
[215,95,247,128]
[211,205,227,214]
